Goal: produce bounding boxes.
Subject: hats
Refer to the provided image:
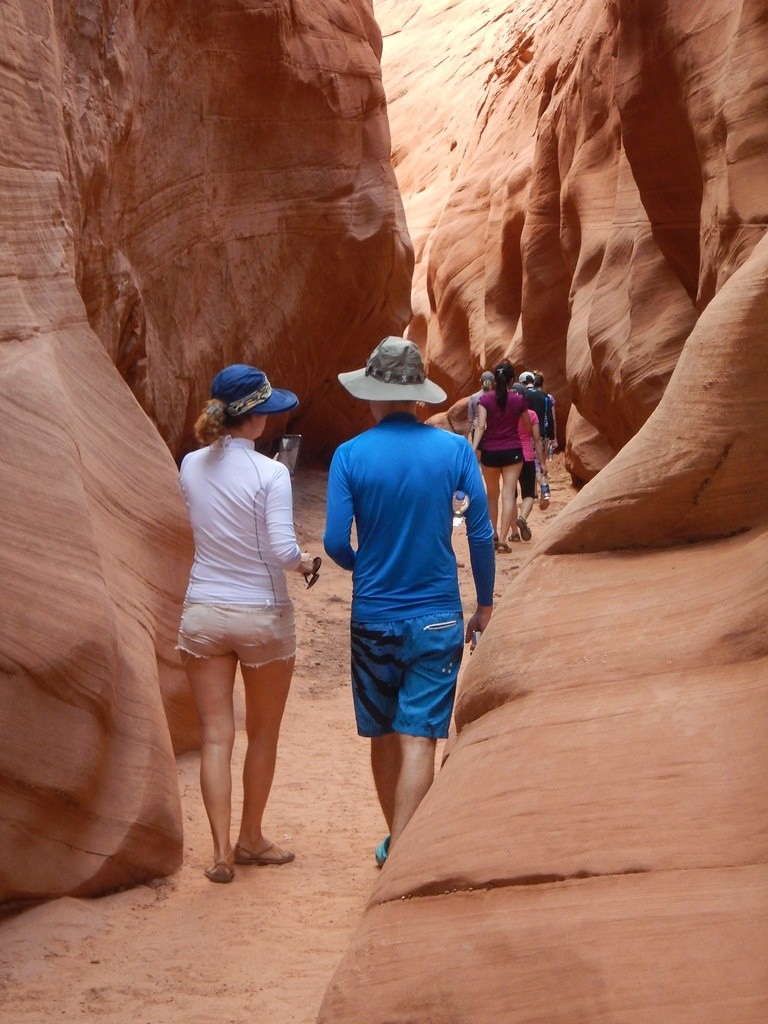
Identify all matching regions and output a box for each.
[211,364,300,416]
[519,371,535,382]
[481,371,495,382]
[338,336,448,403]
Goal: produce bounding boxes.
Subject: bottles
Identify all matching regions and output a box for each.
[540,475,551,499]
[452,490,470,526]
[548,445,553,461]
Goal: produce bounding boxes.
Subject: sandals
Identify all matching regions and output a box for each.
[517,515,531,541]
[204,860,235,883]
[497,543,512,554]
[235,838,295,865]
[508,532,521,542]
[493,538,498,549]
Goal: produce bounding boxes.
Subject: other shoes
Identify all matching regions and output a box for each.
[375,835,392,866]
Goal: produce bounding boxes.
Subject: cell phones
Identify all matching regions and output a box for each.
[277,434,301,481]
[472,631,480,645]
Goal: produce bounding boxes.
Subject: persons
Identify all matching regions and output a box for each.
[323,336,497,867]
[465,357,559,553]
[174,364,315,883]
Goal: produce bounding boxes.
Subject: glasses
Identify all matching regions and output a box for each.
[304,550,322,589]
